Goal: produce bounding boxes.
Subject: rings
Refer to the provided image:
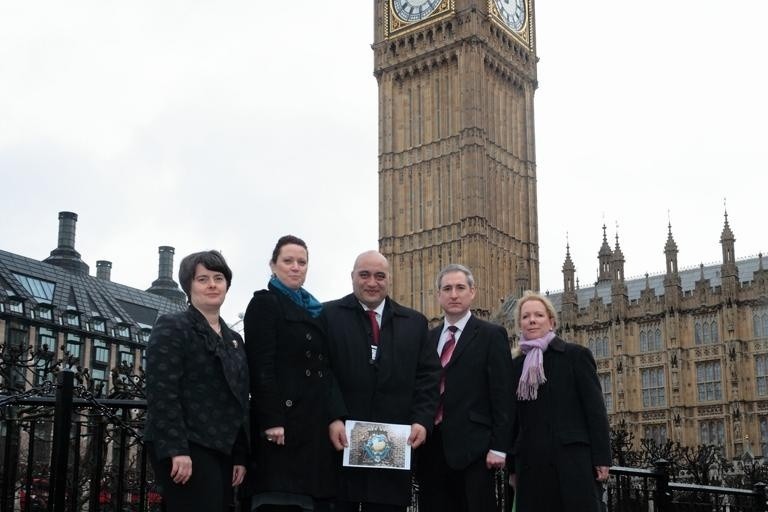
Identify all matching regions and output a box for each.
[267,437,273,441]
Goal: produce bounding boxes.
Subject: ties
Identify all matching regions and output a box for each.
[367,311,380,348]
[434,327,460,424]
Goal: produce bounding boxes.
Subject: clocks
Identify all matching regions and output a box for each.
[387,0,442,24]
[492,0,526,30]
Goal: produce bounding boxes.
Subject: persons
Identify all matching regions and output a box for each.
[243,235,325,511]
[415,264,515,511]
[322,250,442,511]
[142,250,250,511]
[507,293,612,511]
